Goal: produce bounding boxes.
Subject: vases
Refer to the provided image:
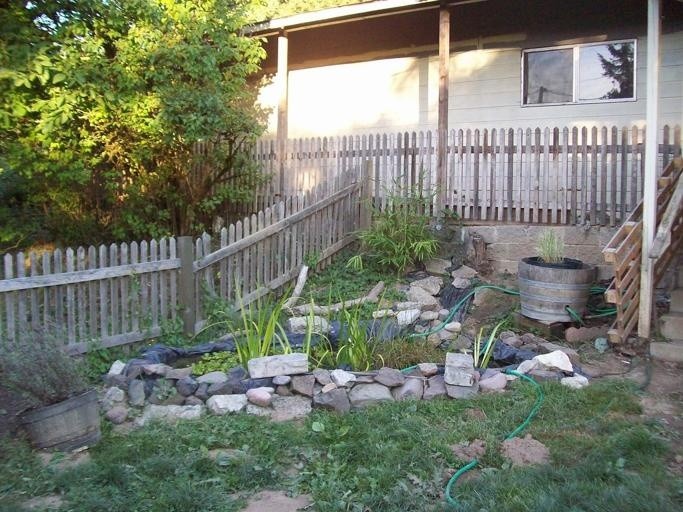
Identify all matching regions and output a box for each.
[17,387,102,455]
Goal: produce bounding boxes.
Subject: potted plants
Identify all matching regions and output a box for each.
[517,227,595,324]
